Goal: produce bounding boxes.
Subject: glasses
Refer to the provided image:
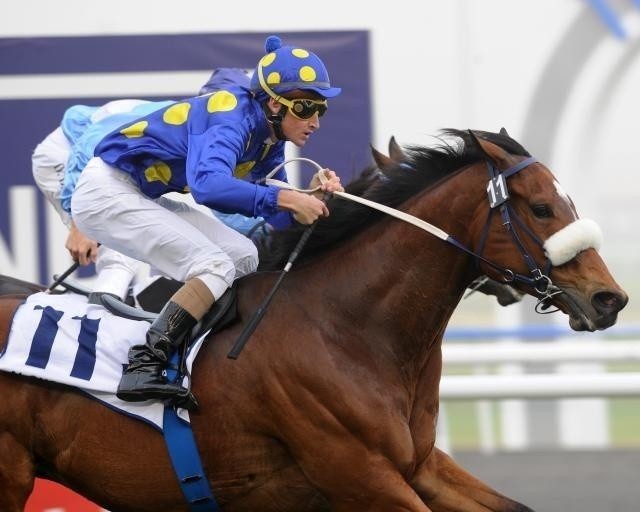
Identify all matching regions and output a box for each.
[257,56,328,121]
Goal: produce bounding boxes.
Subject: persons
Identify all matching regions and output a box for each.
[67,35,350,410]
[30,98,274,321]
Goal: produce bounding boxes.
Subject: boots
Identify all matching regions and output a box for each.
[117,302,198,410]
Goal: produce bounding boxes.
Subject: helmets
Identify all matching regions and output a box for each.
[250,36,341,102]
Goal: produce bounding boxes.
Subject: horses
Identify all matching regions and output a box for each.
[343,135,528,308]
[0,126,629,512]
